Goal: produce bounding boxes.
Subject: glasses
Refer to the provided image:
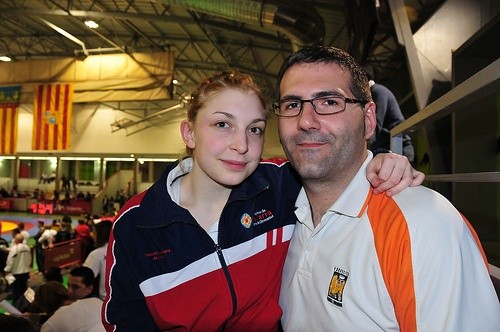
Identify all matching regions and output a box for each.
[272,94,370,118]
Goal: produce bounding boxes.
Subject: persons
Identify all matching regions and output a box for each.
[101,69,425,332]
[271,46,500,332]
[0,170,137,332]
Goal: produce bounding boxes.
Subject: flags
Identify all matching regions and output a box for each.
[0,102,19,156]
[32,82,73,152]
